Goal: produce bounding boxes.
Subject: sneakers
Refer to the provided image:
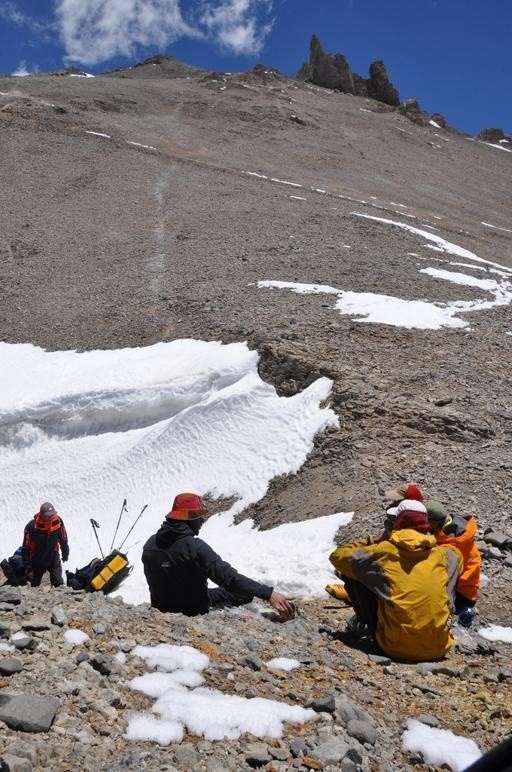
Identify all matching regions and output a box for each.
[325,583,354,606]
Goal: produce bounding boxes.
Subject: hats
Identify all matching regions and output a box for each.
[385,484,448,520]
[166,493,209,521]
[41,503,56,517]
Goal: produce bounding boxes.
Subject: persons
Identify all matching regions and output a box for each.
[20,500,69,588]
[323,484,425,608]
[327,496,467,666]
[423,499,482,617]
[0,544,34,588]
[139,491,298,620]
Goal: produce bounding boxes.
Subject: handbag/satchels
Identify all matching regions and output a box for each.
[0,547,30,586]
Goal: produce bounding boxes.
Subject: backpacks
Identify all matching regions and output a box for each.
[66,550,130,594]
